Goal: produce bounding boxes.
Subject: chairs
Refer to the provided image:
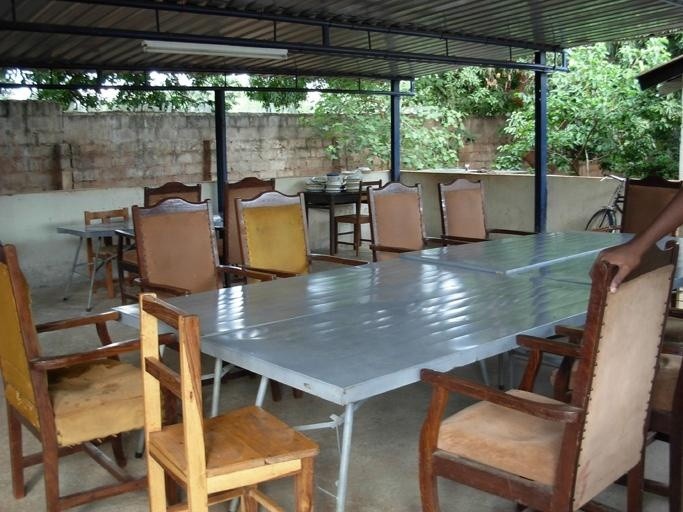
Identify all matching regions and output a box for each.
[333,179,382,255]
[367,180,471,262]
[234,187,370,285]
[84,207,130,299]
[1,242,178,512]
[114,181,202,306]
[131,196,278,299]
[217,175,276,288]
[437,177,536,241]
[620,176,683,239]
[135,292,322,512]
[417,239,682,512]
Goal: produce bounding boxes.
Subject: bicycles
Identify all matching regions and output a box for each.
[584,173,629,233]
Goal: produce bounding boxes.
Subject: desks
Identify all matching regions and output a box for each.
[199,278,592,511]
[296,189,367,253]
[396,228,681,297]
[55,214,223,313]
[111,255,499,460]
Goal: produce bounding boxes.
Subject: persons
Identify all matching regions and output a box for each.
[587,183,683,295]
